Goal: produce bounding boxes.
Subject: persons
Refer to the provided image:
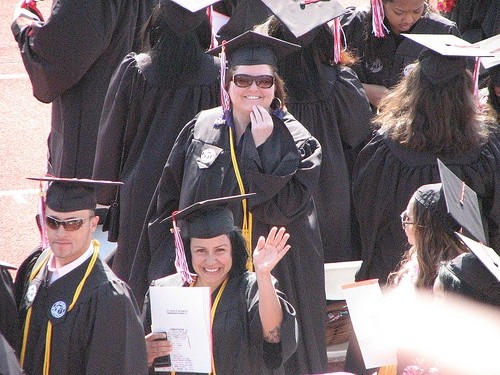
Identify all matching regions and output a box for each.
[330,0,463,113]
[343,33,500,375]
[248,0,375,263]
[0,177,148,375]
[439,0,500,44]
[210,0,371,62]
[142,193,299,375]
[473,35,500,115]
[128,30,328,375]
[396,231,500,375]
[379,158,487,291]
[11,0,158,224]
[91,0,228,313]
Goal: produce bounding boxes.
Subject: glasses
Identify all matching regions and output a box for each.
[43,215,94,231]
[232,74,275,89]
[401,211,415,228]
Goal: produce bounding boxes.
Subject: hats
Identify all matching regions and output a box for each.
[158,0,389,64]
[160,192,257,240]
[446,231,500,301]
[415,158,486,245]
[401,34,495,86]
[25,177,124,212]
[204,31,301,65]
[472,34,500,69]
